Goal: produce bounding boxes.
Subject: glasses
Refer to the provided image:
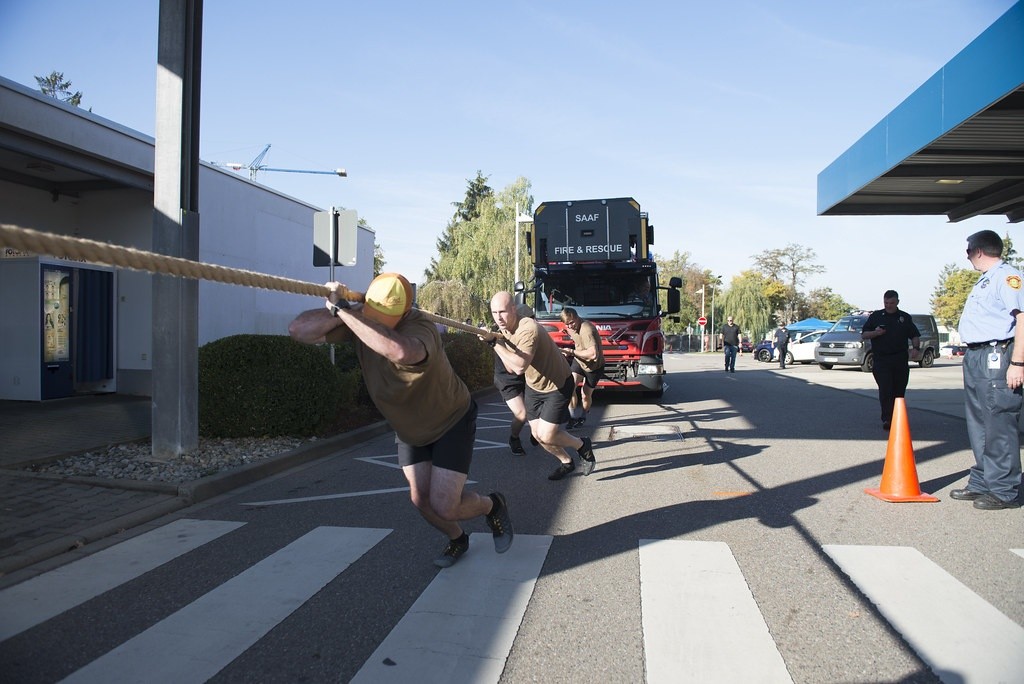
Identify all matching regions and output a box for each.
[727,320,732,322]
[967,249,973,255]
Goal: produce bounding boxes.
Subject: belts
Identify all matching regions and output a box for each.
[968,337,1014,350]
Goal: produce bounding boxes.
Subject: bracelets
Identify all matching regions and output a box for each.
[913,346,920,351]
[1010,360,1024,366]
[739,341,742,344]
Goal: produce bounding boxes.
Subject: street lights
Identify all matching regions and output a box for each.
[694,285,705,352]
[514,201,534,295]
[711,274,722,352]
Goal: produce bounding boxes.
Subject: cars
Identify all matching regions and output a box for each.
[737,338,753,352]
[753,340,778,363]
[773,330,828,365]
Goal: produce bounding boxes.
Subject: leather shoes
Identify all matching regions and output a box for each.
[950,487,987,500]
[973,494,1020,510]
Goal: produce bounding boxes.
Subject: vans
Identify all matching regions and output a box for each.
[814,314,940,373]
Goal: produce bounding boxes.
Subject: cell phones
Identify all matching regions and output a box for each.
[880,325,885,330]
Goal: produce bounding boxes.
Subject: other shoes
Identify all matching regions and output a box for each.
[884,420,891,430]
[725,367,734,373]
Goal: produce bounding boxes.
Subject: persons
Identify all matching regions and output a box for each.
[466,318,472,325]
[289,271,512,569]
[861,290,922,428]
[560,307,606,429]
[477,291,597,481]
[771,322,792,369]
[478,319,486,327]
[623,280,653,305]
[435,322,448,334]
[492,304,538,456]
[950,230,1024,510]
[720,317,743,372]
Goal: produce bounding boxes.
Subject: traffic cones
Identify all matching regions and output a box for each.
[864,399,940,504]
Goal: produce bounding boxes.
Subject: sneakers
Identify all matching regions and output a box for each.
[486,492,513,553]
[530,434,539,447]
[566,418,578,429]
[548,458,575,480]
[508,435,524,456]
[576,437,596,476]
[432,534,469,568]
[574,417,586,428]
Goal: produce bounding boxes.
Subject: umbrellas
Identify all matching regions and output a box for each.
[785,317,834,331]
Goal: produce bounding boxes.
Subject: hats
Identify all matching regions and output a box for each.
[779,322,785,326]
[515,304,534,318]
[362,273,413,330]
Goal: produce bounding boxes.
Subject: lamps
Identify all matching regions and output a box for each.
[27,163,57,176]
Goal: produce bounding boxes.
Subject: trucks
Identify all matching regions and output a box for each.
[514,197,683,401]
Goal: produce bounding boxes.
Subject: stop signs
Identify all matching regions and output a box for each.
[698,317,707,326]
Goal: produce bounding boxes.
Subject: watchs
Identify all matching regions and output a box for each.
[330,299,351,317]
